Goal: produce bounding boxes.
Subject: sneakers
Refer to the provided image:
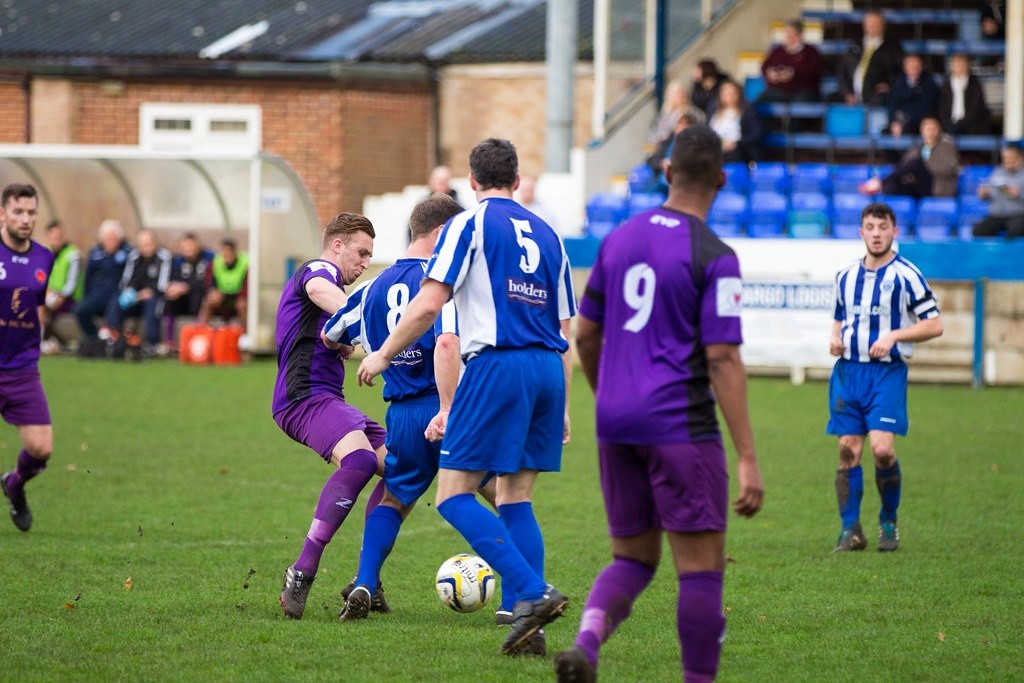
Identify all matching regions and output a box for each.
[1,472,33,532]
[280,562,314,620]
[516,628,546,655]
[339,585,372,622]
[555,647,596,683]
[494,606,515,624]
[342,574,389,612]
[834,525,868,554]
[501,584,569,655]
[878,522,900,551]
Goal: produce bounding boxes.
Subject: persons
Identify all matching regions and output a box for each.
[973,143,1024,236]
[272,213,391,620]
[825,0,1008,135]
[826,203,943,551]
[858,119,957,196]
[409,164,461,241]
[0,183,55,531]
[357,136,578,655]
[756,19,823,103]
[647,60,766,192]
[322,192,519,622]
[38,219,248,360]
[555,124,763,683]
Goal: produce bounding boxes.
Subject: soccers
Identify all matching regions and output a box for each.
[436,553,496,613]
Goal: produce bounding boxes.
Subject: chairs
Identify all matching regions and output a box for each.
[581,12,1024,239]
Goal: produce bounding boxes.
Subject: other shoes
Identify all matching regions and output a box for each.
[139,344,168,360]
[111,337,126,358]
[79,335,97,357]
[41,340,60,355]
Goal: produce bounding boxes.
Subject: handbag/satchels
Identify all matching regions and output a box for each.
[880,158,934,199]
